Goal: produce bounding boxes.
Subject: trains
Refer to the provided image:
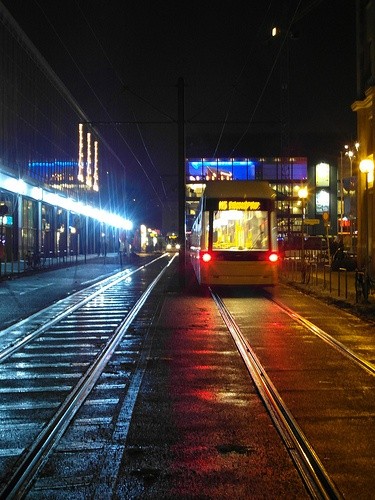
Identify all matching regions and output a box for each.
[187,180,278,289]
[165,235,180,252]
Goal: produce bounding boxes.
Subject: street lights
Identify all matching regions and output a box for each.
[359,159,374,277]
[344,143,359,177]
[298,189,308,283]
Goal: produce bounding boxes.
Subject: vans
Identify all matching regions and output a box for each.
[343,251,357,262]
